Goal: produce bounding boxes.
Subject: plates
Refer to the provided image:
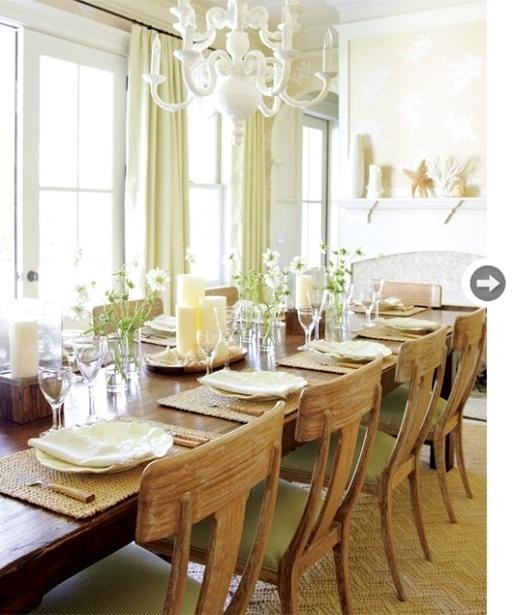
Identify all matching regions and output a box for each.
[205,370,310,402]
[383,322,434,334]
[34,421,175,474]
[308,340,393,363]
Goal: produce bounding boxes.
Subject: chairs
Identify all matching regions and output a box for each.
[0,273,487,614]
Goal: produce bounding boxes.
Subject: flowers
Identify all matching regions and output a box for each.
[222,247,306,330]
[66,258,173,383]
[309,241,366,323]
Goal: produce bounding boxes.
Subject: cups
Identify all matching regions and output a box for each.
[241,297,287,353]
[104,325,141,393]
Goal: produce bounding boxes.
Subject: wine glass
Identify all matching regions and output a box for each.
[197,328,221,381]
[213,306,240,372]
[358,278,386,328]
[36,333,107,438]
[295,289,327,351]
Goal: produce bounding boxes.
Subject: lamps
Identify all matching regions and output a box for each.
[140,0,338,148]
